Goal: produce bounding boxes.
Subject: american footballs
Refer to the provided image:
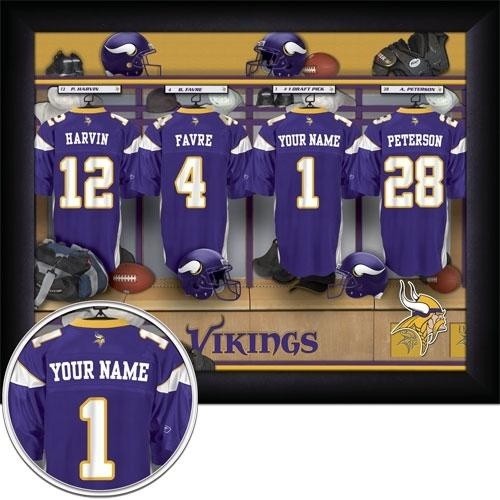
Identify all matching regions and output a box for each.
[426,266,461,293]
[109,263,155,294]
[302,53,339,77]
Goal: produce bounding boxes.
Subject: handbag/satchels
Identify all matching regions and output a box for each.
[34,239,108,307]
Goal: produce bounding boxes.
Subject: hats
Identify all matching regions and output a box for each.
[208,87,242,115]
[35,86,88,124]
[302,88,345,113]
[145,88,179,113]
[420,86,465,120]
[289,271,335,295]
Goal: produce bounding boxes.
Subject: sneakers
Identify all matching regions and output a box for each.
[276,92,294,108]
[256,88,275,109]
[44,50,83,77]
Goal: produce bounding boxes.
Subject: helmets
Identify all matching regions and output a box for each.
[325,251,387,301]
[175,249,242,302]
[101,31,162,76]
[245,31,307,78]
